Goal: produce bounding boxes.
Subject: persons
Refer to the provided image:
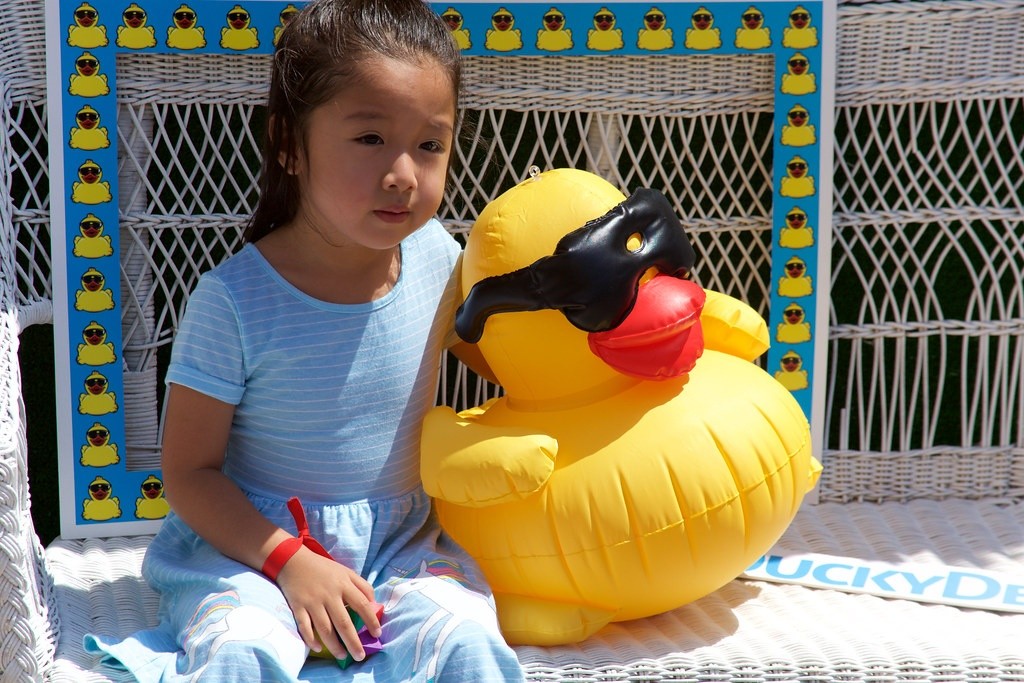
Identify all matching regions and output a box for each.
[87,0,529,683]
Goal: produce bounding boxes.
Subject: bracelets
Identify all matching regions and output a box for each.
[262,494,336,583]
